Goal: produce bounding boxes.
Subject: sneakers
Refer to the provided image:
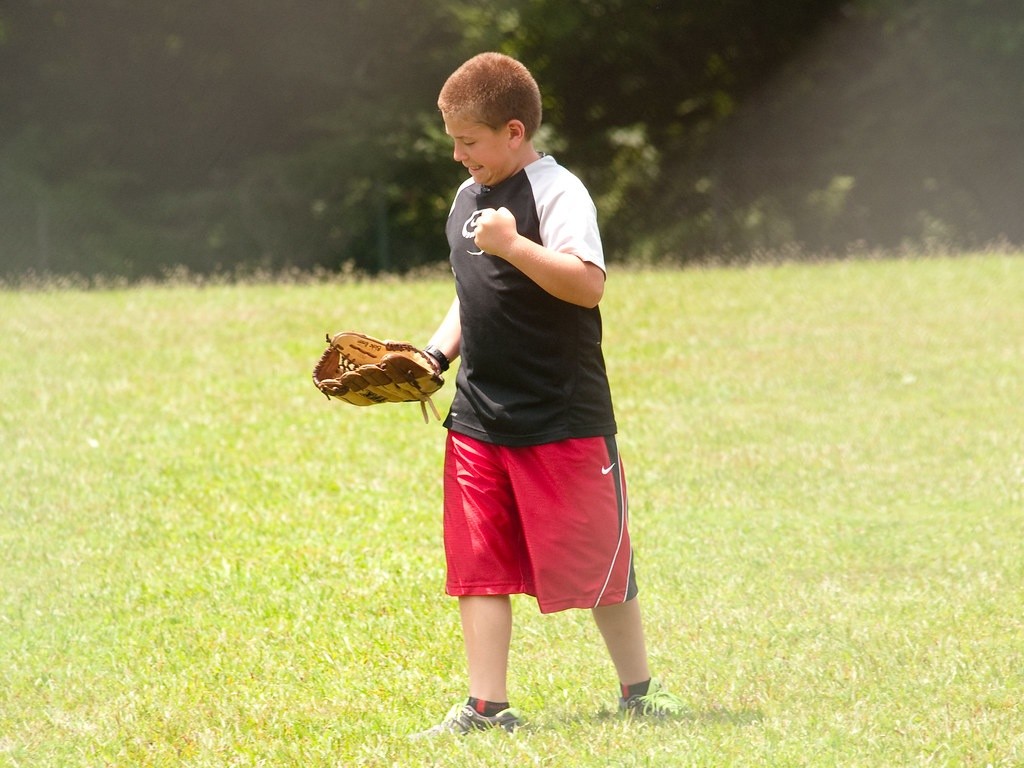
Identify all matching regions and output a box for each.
[619,679,680,722]
[410,699,519,743]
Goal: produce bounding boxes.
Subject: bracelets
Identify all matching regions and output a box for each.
[423,345,449,374]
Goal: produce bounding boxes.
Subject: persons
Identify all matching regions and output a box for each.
[311,52,652,734]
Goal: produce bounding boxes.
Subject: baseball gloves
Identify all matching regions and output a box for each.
[312,331,445,406]
